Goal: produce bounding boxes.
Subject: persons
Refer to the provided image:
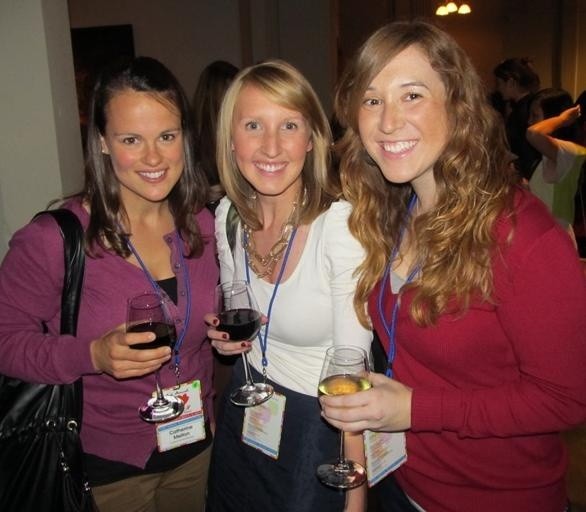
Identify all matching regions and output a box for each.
[487,89,515,124]
[516,85,586,257]
[489,55,543,183]
[0,51,224,512]
[202,58,375,512]
[316,12,586,512]
[188,58,239,215]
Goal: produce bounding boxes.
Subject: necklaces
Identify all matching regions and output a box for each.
[240,197,300,281]
[236,192,304,266]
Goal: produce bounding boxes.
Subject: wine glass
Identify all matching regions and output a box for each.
[315,345,372,490]
[126,291,185,424]
[213,279,274,407]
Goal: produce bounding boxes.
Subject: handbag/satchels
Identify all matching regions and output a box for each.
[0,374,96,511]
